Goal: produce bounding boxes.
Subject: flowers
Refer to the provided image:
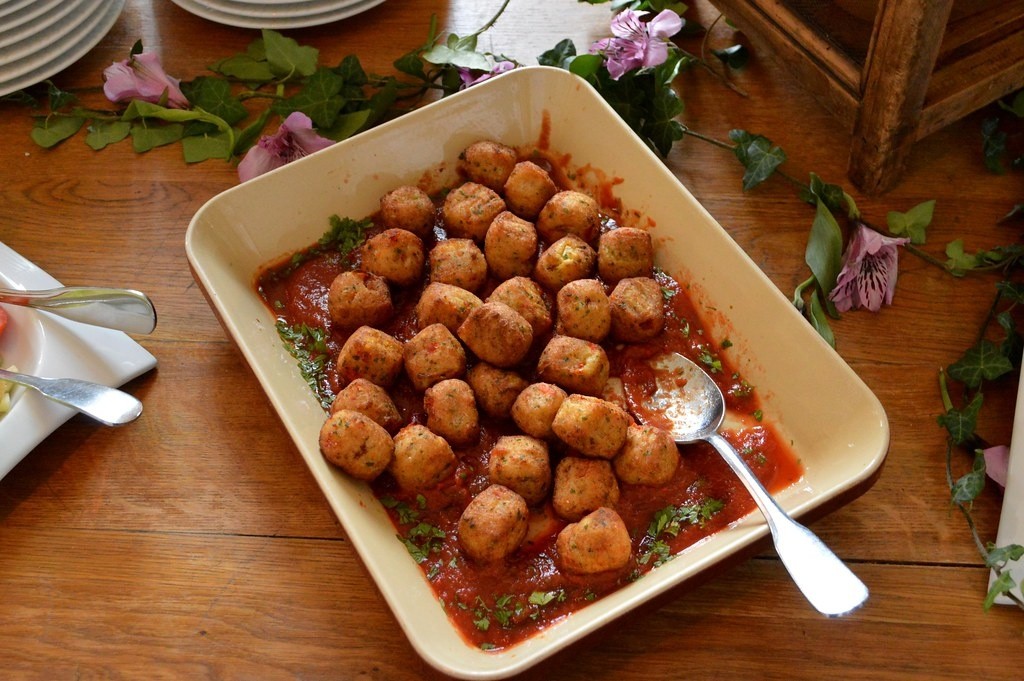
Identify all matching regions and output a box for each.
[39,10,997,343]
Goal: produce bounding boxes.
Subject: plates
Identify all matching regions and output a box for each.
[0,241,157,481]
[171,0,387,28]
[185,65,891,680]
[0,0,127,95]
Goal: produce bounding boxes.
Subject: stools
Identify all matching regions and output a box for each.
[707,0,1023,199]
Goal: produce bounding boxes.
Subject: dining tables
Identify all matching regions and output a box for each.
[0,0,1024,681]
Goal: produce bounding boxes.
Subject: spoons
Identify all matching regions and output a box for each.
[618,353,870,618]
